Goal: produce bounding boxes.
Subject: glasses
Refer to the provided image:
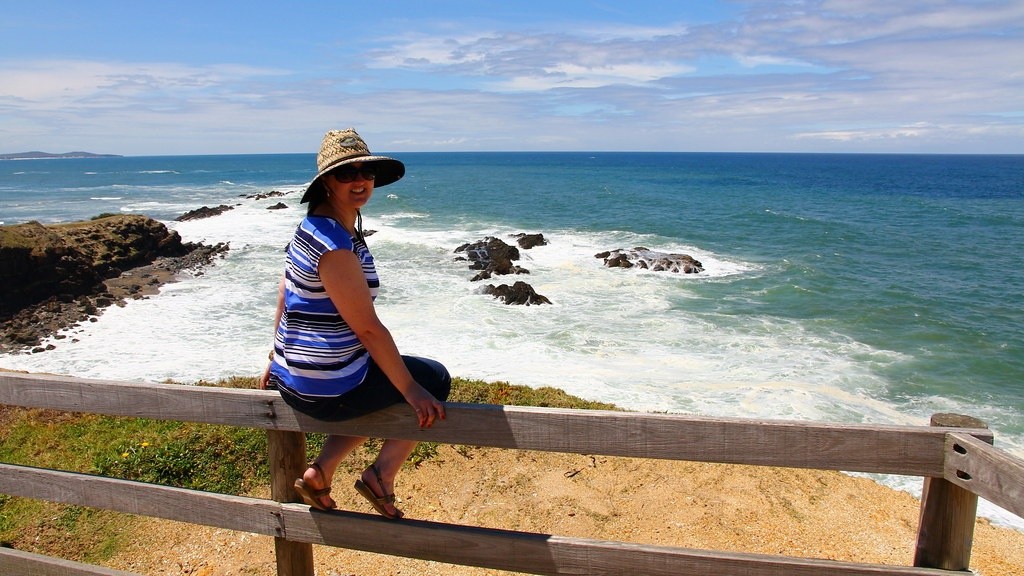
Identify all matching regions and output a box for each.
[327,163,378,183]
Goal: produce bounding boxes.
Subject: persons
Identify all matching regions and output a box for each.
[260,127,451,518]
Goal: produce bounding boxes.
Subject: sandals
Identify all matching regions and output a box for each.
[294,463,337,511]
[354,464,404,520]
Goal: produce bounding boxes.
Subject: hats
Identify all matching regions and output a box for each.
[299,127,405,204]
